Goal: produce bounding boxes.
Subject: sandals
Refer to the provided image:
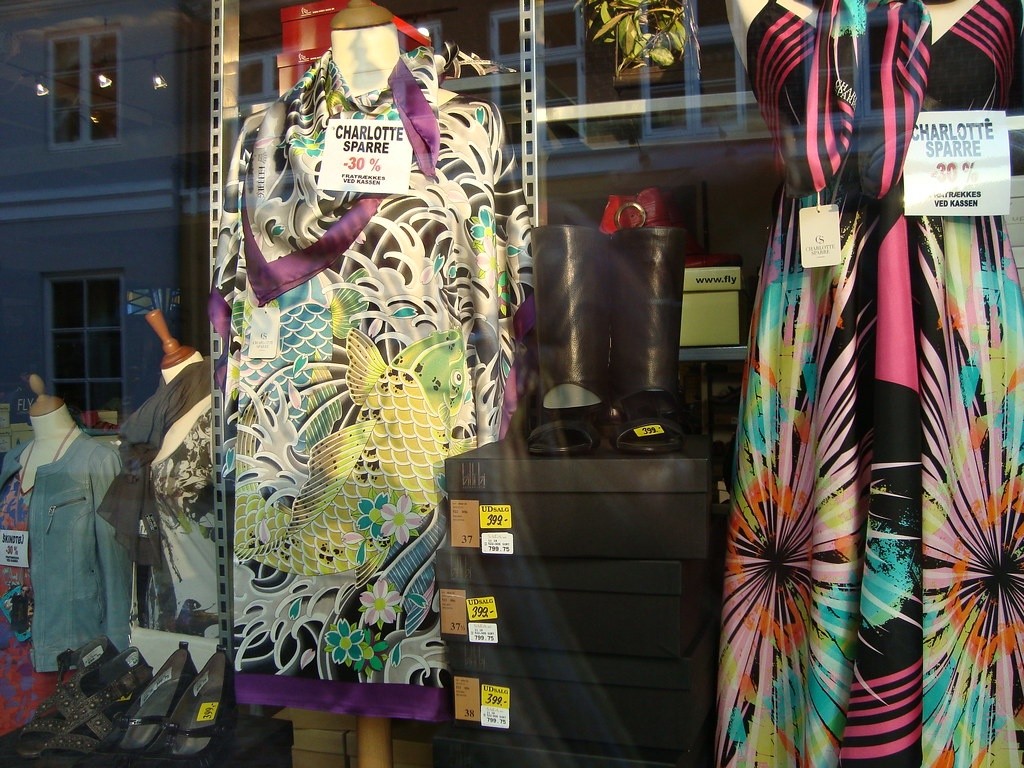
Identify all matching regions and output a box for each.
[17,634,153,768]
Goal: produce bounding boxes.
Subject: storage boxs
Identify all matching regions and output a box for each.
[434,415,730,768]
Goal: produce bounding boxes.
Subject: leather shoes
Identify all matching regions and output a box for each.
[76,641,239,768]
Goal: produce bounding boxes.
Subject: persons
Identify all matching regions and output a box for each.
[715,0,1024,767]
[206,6,535,723]
[0,395,133,735]
[93,347,212,637]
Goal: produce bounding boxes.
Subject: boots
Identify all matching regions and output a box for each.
[609,224,685,456]
[526,220,606,457]
